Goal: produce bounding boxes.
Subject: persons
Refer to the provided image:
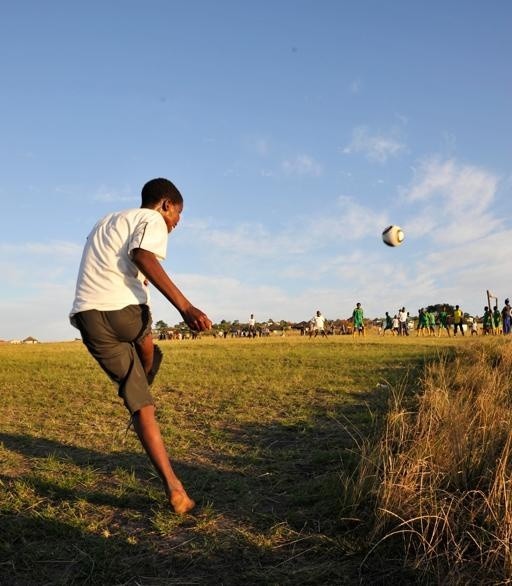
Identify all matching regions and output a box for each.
[247,314,255,327]
[70,177,214,514]
[312,311,328,338]
[353,303,365,335]
[382,298,511,337]
[157,320,273,341]
[298,320,356,336]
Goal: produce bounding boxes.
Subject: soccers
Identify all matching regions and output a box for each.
[382,225,405,248]
